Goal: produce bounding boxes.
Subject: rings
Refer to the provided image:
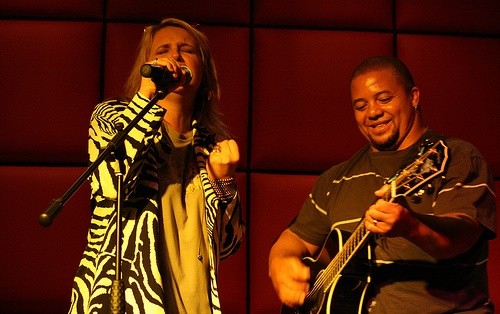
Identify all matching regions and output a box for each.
[213,145,221,153]
[373,219,379,226]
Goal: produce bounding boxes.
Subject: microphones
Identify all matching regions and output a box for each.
[140,64,193,85]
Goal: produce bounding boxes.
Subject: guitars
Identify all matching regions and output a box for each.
[280,137,449,314]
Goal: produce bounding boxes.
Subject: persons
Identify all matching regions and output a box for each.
[269,54,496,314]
[67,19,245,314]
